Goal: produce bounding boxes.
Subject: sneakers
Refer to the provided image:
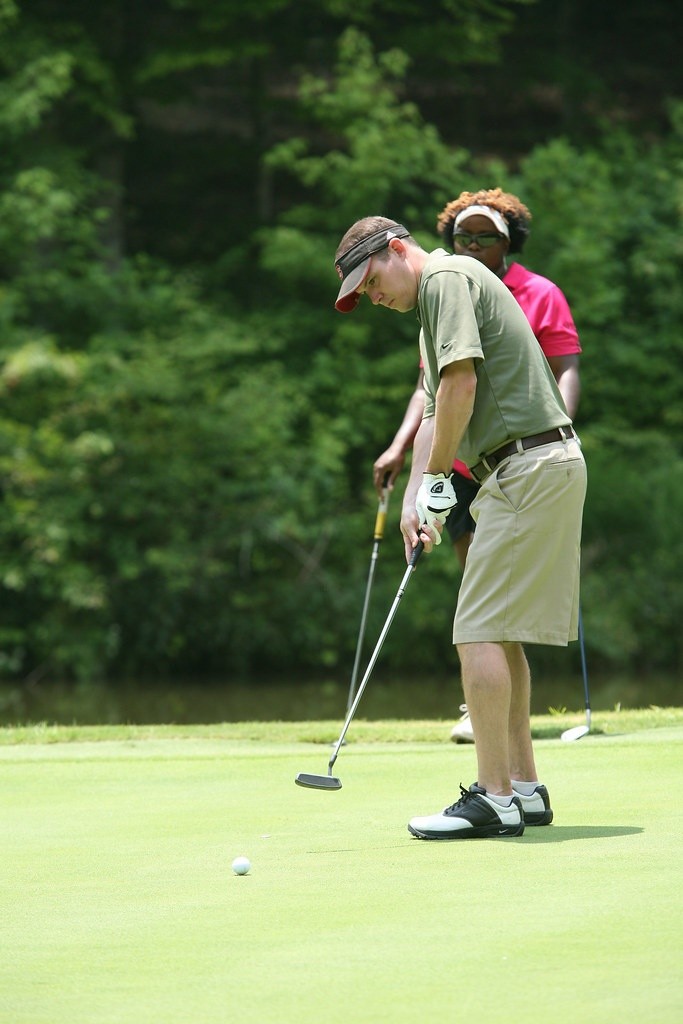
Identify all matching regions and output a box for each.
[451,704,475,744]
[408,782,553,839]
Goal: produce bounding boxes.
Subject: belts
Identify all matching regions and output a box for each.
[470,425,574,483]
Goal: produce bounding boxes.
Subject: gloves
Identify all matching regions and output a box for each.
[415,471,457,545]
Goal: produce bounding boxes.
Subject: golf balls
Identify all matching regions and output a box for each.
[232,856,250,875]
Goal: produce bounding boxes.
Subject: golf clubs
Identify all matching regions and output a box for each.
[294,524,427,793]
[556,606,593,744]
[329,481,392,747]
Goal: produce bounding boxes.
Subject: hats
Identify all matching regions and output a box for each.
[335,224,410,313]
[453,205,510,241]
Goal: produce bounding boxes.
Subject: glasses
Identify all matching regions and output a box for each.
[452,232,506,248]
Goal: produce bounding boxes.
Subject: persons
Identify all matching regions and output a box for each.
[375,189,583,743]
[336,216,587,839]
